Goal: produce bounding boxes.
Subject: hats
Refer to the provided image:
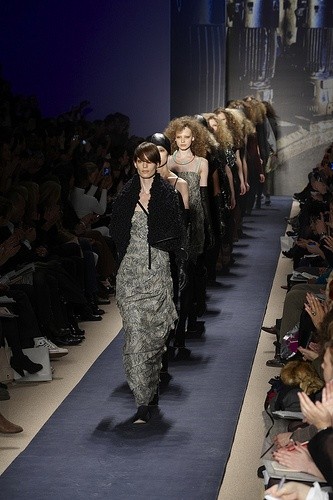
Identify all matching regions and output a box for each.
[150,133,171,153]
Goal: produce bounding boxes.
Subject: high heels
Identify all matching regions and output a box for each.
[10,356,42,376]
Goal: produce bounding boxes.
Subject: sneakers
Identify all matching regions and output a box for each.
[34,338,68,357]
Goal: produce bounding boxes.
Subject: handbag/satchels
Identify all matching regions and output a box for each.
[13,348,52,383]
[264,378,316,415]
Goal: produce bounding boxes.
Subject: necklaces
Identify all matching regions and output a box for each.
[172,149,195,165]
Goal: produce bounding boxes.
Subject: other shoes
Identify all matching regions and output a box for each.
[293,192,305,201]
[267,360,281,366]
[0,415,23,434]
[287,231,295,235]
[282,250,288,256]
[286,216,300,225]
[93,308,104,315]
[261,327,277,334]
[48,314,86,345]
[133,407,151,423]
[160,373,171,390]
[281,286,290,290]
[147,400,160,418]
[98,298,110,303]
[106,284,114,292]
[0,383,15,399]
[83,313,101,320]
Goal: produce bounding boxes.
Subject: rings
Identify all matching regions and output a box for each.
[312,311,317,316]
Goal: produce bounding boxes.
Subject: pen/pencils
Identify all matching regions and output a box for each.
[288,441,309,451]
[278,475,286,490]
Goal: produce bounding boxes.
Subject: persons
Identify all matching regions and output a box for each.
[260,140,333,500]
[162,94,278,336]
[103,141,188,423]
[148,131,190,359]
[0,74,148,435]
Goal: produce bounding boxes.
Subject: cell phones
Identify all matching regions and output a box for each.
[104,167,110,176]
